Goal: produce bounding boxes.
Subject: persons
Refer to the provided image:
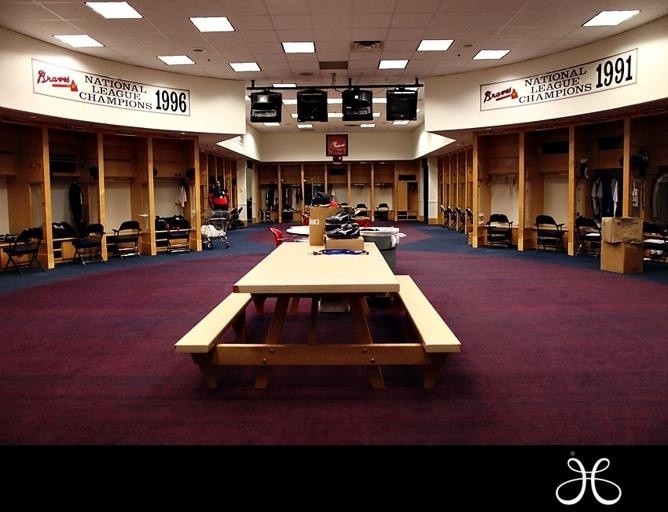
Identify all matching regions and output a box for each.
[208,180,231,218]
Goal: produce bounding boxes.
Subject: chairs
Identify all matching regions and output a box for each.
[3,228,45,277]
[112,220,143,261]
[166,219,190,256]
[440,205,473,233]
[270,227,289,249]
[342,202,390,222]
[643,221,668,272]
[534,214,565,256]
[574,214,602,262]
[487,214,513,248]
[72,224,104,267]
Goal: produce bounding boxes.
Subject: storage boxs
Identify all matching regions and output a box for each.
[600,216,643,244]
[601,240,643,275]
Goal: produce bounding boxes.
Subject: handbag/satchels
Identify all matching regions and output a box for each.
[310,192,332,206]
[170,214,187,228]
[52,220,75,239]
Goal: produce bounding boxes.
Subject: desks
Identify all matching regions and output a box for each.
[233,241,400,390]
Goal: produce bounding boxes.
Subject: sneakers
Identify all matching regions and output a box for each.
[325,207,356,223]
[326,223,360,239]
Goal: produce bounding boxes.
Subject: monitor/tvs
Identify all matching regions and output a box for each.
[251,89,282,123]
[296,88,328,123]
[342,88,373,121]
[386,87,417,121]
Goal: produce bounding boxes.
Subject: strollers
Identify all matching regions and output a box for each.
[201,216,231,250]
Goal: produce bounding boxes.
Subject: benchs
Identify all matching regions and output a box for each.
[173,263,268,390]
[393,275,461,388]
